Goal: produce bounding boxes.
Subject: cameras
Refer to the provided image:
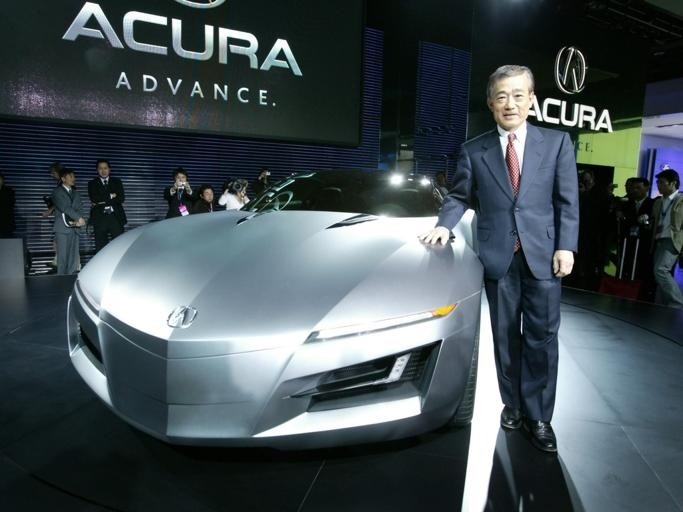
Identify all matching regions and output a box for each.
[176,181,187,189]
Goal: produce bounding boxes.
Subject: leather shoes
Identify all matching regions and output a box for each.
[500,404,524,429]
[522,417,558,453]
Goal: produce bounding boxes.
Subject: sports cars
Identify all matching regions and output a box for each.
[66,165,484,448]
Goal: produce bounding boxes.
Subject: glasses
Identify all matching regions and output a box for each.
[656,180,673,184]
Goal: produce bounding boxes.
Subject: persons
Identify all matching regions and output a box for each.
[576,168,634,293]
[435,169,453,192]
[218,176,251,211]
[643,169,683,312]
[88,160,128,252]
[164,167,193,218]
[191,185,218,215]
[1,171,16,238]
[40,162,77,275]
[415,64,581,453]
[49,168,86,277]
[252,168,271,195]
[615,177,657,303]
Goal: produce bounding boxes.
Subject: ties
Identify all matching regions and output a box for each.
[505,133,522,253]
[179,189,183,202]
[104,180,108,191]
